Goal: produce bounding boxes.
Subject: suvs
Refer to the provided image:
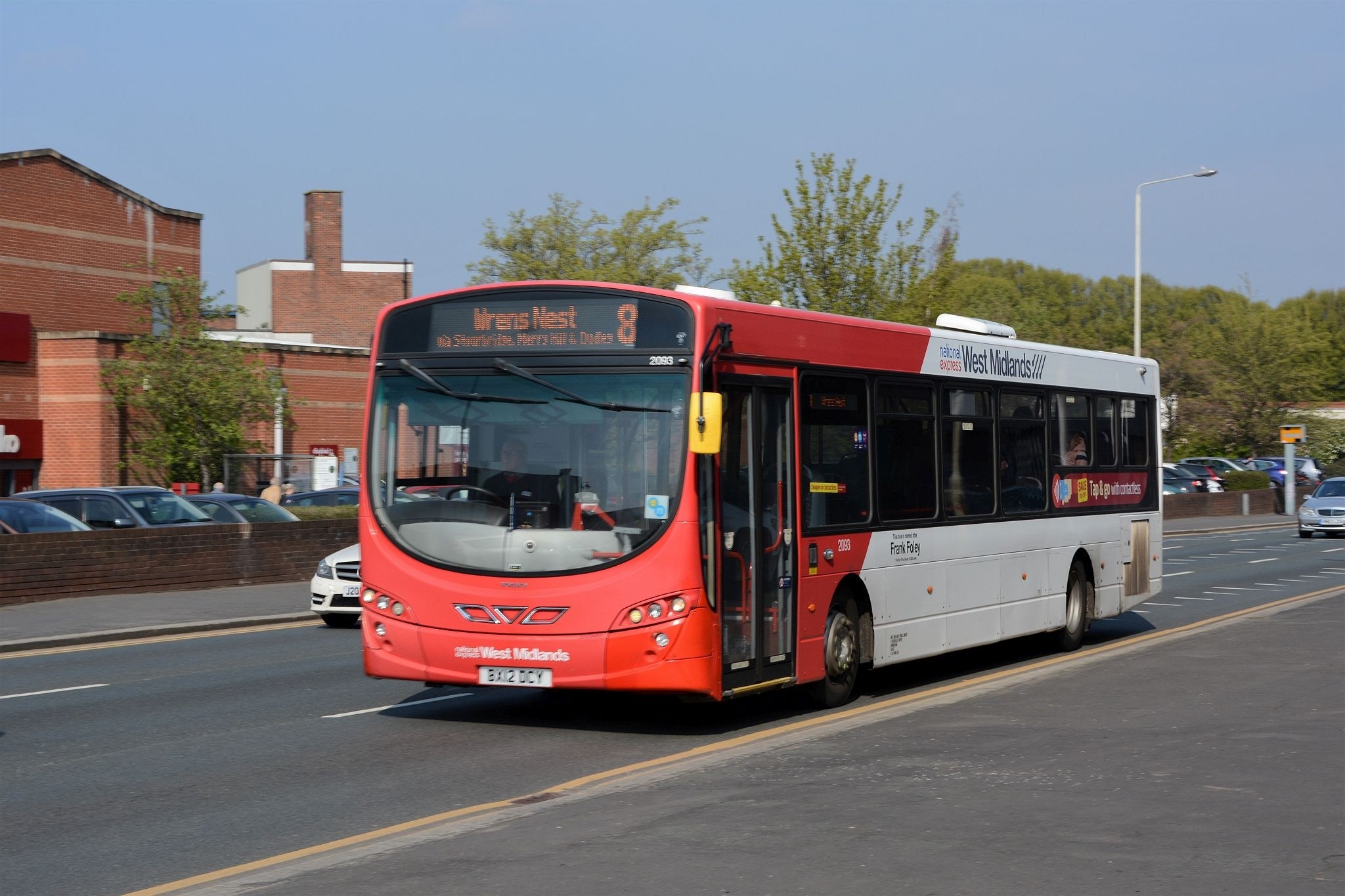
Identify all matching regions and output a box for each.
[10,486,217,529]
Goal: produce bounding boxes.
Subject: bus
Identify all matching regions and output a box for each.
[302,279,1164,713]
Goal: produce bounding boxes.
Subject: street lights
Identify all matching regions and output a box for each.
[1133,167,1220,359]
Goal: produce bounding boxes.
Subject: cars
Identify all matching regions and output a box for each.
[173,493,301,522]
[309,542,364,629]
[279,472,504,507]
[1297,475,1345,539]
[0,496,100,534]
[1163,456,1324,496]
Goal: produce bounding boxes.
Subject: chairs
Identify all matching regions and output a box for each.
[825,426,1132,523]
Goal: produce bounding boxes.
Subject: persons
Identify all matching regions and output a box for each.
[210,482,226,494]
[258,476,296,505]
[1242,455,1257,470]
[479,438,540,503]
[855,420,1088,516]
